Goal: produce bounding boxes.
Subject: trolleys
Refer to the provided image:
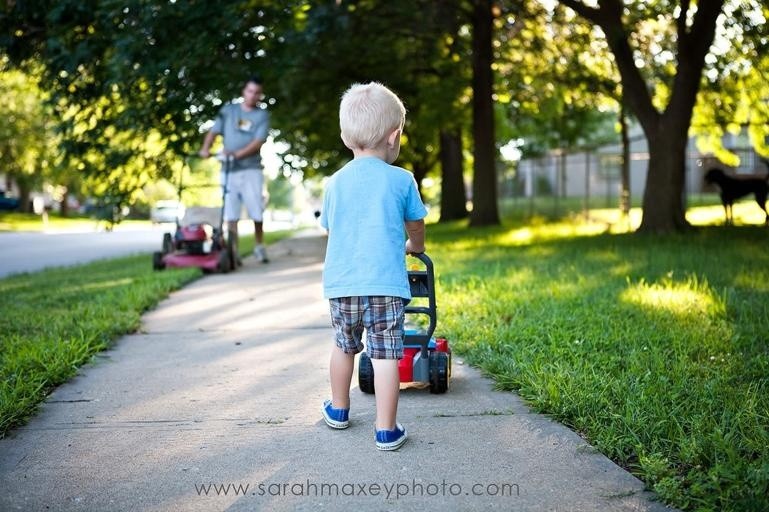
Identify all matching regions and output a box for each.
[151,151,239,274]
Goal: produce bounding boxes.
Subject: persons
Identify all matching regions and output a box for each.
[192,79,274,269]
[318,79,429,453]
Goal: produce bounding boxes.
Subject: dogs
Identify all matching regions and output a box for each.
[702,165,769,224]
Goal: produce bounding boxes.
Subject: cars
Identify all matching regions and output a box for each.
[148,197,186,224]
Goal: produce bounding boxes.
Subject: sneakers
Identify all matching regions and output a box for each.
[320,398,350,430]
[373,418,408,451]
[253,243,269,264]
